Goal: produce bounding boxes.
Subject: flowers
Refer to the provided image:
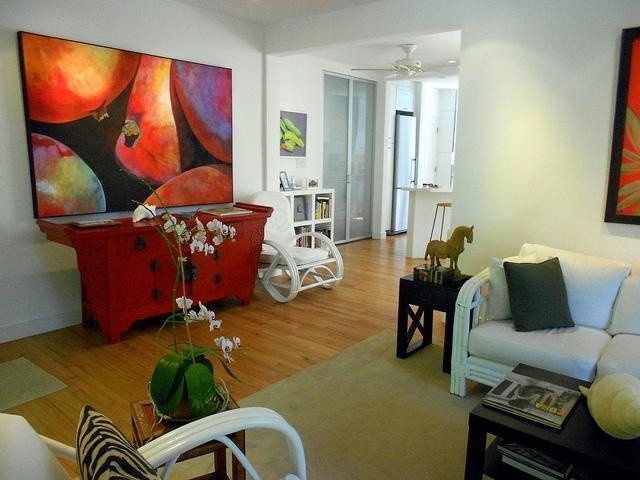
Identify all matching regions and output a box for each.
[116,167,240,414]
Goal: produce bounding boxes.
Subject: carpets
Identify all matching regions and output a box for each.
[157,327,501,479]
[0,357,67,409]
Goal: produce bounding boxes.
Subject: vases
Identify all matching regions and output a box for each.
[146,373,230,442]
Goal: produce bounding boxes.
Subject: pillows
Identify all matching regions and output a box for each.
[75,404,160,480]
[503,258,576,331]
[490,257,514,321]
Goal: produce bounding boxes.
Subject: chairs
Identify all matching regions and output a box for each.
[244,190,344,304]
[0,408,309,479]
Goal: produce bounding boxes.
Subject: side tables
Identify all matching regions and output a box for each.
[129,379,246,480]
[397,266,473,374]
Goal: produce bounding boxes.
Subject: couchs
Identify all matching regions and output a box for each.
[449,240,640,397]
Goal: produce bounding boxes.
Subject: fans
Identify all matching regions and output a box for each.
[351,43,461,79]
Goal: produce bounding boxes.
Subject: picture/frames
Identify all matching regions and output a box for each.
[604,27,640,225]
[280,172,292,189]
[16,30,233,218]
[280,109,309,159]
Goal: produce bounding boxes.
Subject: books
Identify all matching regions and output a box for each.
[481,371,582,431]
[496,437,573,480]
[199,207,253,216]
[296,195,331,248]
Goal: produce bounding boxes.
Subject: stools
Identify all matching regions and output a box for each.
[424,203,452,259]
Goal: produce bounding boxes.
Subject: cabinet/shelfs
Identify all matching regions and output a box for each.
[33,202,274,344]
[280,188,334,251]
